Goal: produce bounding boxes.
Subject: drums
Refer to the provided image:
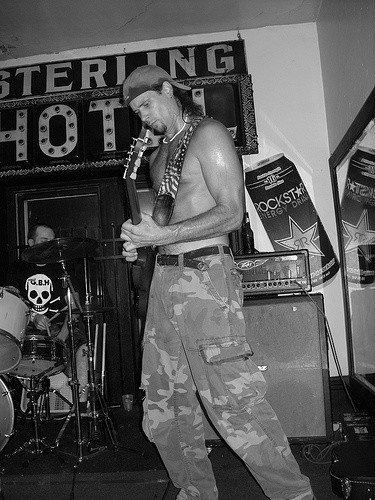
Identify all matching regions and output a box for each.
[8,334,68,378]
[0,286,31,376]
[0,378,18,453]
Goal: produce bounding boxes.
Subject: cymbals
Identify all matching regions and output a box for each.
[56,305,120,314]
[20,236,99,264]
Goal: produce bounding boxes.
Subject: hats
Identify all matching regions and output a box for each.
[123,65,192,105]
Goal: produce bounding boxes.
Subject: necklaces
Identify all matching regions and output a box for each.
[162,114,187,144]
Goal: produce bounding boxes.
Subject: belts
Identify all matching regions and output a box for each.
[157,245,230,271]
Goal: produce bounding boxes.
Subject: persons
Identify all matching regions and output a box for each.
[0,223,91,414]
[120,67,317,500]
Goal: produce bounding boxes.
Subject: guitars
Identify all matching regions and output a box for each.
[122,122,166,319]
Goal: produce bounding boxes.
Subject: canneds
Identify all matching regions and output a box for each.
[339,146,375,290]
[245,152,340,291]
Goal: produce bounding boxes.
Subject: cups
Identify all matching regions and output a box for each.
[122,394,133,412]
